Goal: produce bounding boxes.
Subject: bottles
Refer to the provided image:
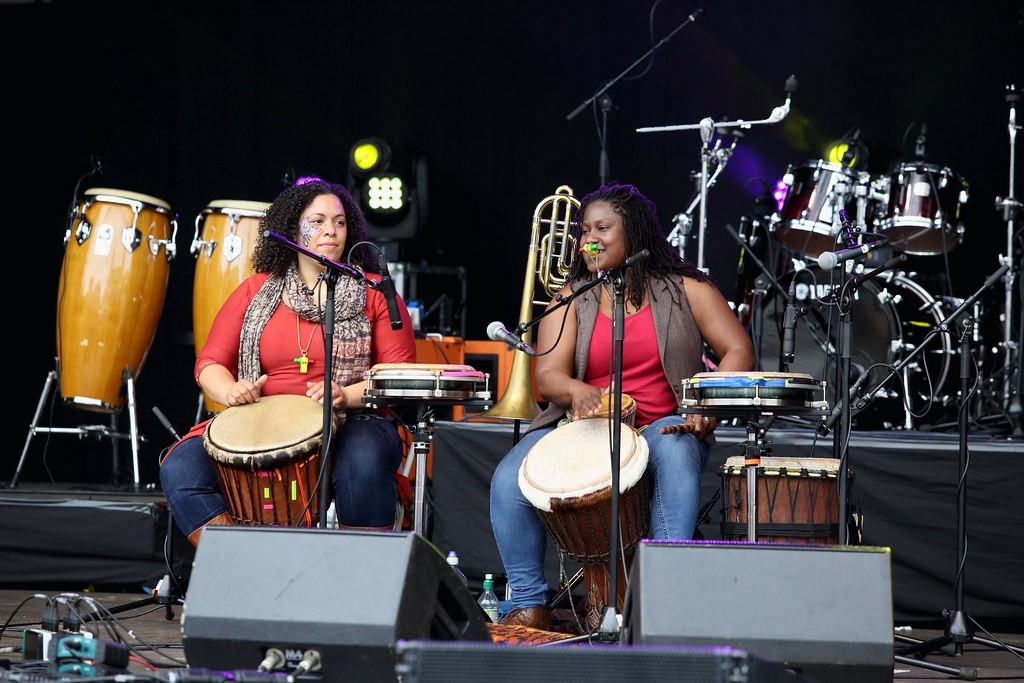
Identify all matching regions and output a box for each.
[477,574,499,624]
[446,551,469,590]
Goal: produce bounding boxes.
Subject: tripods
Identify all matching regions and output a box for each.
[79,508,186,623]
[848,264,1024,660]
[919,273,1024,437]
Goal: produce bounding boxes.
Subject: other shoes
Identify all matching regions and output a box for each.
[499,603,552,634]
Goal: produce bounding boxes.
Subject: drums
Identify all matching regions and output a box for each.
[55,188,174,414]
[691,371,816,408]
[565,392,638,428]
[748,263,952,431]
[518,419,651,632]
[202,393,338,527]
[775,157,885,267]
[368,365,479,396]
[723,455,856,544]
[875,161,971,255]
[193,198,273,412]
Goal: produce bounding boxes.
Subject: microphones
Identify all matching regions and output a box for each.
[915,122,928,156]
[378,254,403,331]
[817,239,887,271]
[849,128,862,146]
[486,320,536,355]
[782,281,796,364]
[815,369,870,438]
[845,362,864,384]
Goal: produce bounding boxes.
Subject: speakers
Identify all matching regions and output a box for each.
[180,524,895,683]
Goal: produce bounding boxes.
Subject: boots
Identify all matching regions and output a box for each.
[186,510,237,549]
[339,522,392,533]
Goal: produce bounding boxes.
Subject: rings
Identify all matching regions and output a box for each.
[704,417,709,420]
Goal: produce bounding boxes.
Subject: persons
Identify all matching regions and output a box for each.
[488,185,756,631]
[158,180,417,548]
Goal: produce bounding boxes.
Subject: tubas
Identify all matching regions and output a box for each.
[482,185,582,419]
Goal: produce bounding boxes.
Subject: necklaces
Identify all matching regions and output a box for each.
[601,285,626,304]
[293,314,319,374]
